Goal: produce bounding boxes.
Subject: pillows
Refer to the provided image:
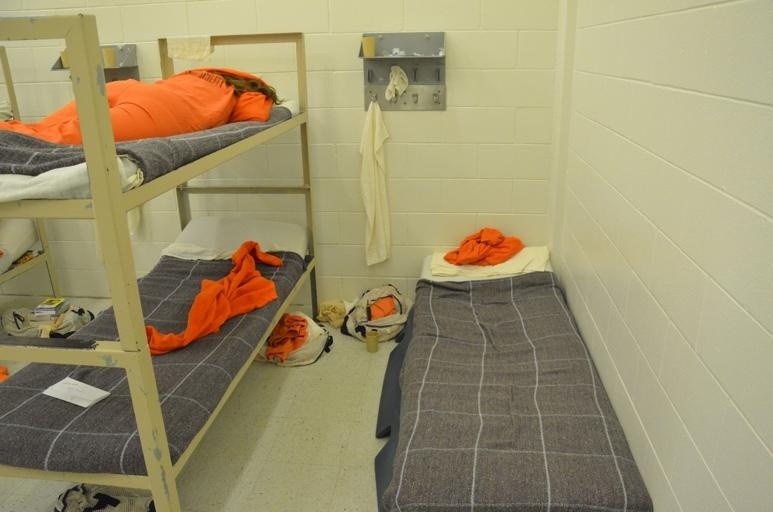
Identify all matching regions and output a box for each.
[164,214,305,259]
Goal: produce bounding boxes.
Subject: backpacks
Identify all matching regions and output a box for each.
[341,283,410,343]
[54,485,155,512]
[3,306,94,338]
[253,312,330,367]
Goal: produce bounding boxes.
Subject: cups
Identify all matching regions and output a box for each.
[366,331,378,353]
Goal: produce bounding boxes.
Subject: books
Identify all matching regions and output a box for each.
[34,298,66,315]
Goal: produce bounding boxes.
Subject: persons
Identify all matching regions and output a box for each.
[0,66,286,148]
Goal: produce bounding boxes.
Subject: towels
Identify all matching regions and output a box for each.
[430,247,549,277]
[384,66,408,101]
[357,100,392,267]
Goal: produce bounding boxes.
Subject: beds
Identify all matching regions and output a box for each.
[0,15,313,512]
[375,252,652,510]
